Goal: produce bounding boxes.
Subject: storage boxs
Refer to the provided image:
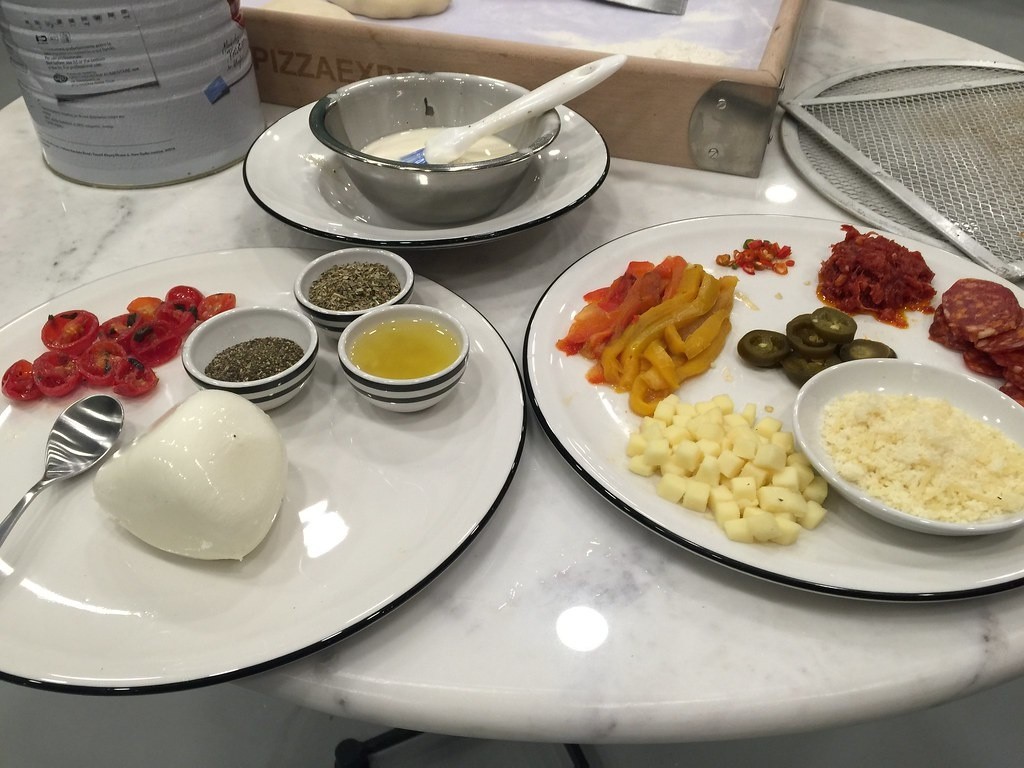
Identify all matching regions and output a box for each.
[246,2,810,179]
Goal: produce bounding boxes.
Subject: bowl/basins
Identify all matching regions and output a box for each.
[182,304,319,412]
[293,247,416,339]
[307,71,561,226]
[337,304,470,413]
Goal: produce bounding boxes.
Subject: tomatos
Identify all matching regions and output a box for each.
[3,285,238,402]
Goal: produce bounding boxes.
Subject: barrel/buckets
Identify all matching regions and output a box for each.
[1,0,265,189]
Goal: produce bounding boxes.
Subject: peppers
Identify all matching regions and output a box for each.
[735,307,898,387]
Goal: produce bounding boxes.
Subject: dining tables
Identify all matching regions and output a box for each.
[1,2,1022,768]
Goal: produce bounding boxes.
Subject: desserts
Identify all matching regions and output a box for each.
[93,387,286,562]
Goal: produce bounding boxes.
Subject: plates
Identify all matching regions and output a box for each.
[794,358,1023,536]
[243,99,610,249]
[523,213,1024,604]
[1,246,527,697]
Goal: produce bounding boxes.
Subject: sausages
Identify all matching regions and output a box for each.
[928,277,1024,409]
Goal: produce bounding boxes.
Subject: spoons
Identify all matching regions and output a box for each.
[0,393,124,549]
